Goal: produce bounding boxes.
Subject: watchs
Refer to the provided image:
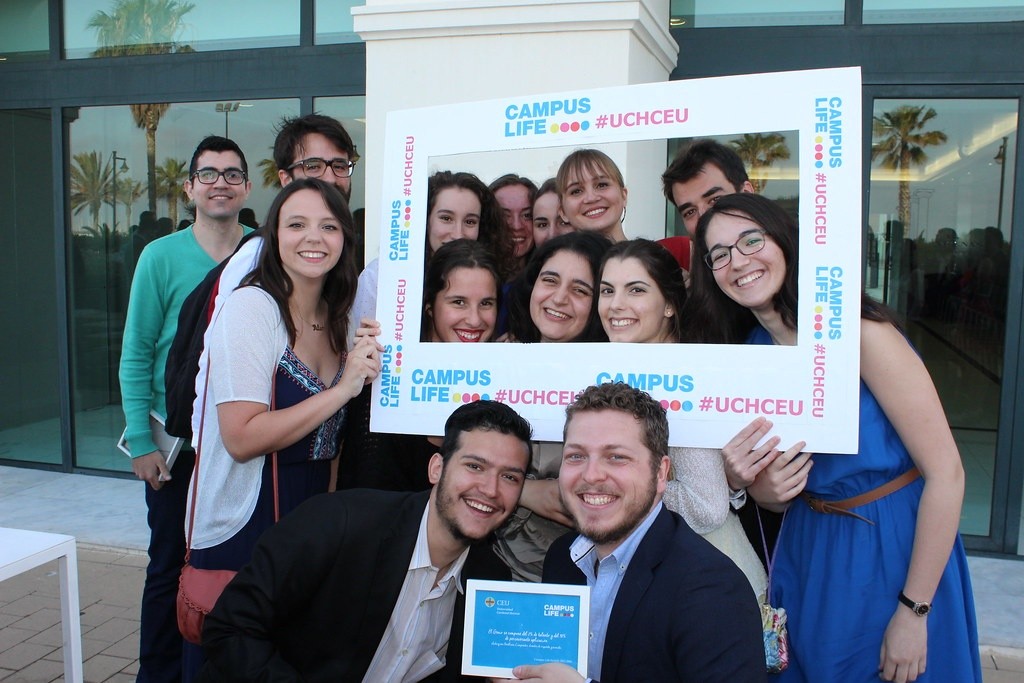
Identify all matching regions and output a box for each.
[897,589,931,616]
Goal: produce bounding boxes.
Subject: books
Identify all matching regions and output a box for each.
[116,407,184,482]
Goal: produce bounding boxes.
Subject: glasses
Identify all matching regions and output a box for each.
[287,157,356,178]
[702,228,767,271]
[189,166,246,185]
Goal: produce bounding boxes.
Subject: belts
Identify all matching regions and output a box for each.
[801,464,922,527]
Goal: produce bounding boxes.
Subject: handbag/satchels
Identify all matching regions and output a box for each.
[174,562,241,646]
[757,604,789,672]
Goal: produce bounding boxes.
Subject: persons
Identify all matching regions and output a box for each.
[676,192,979,681]
[170,399,543,683]
[501,383,770,681]
[116,112,770,683]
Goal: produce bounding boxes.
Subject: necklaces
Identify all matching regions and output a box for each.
[288,304,328,331]
